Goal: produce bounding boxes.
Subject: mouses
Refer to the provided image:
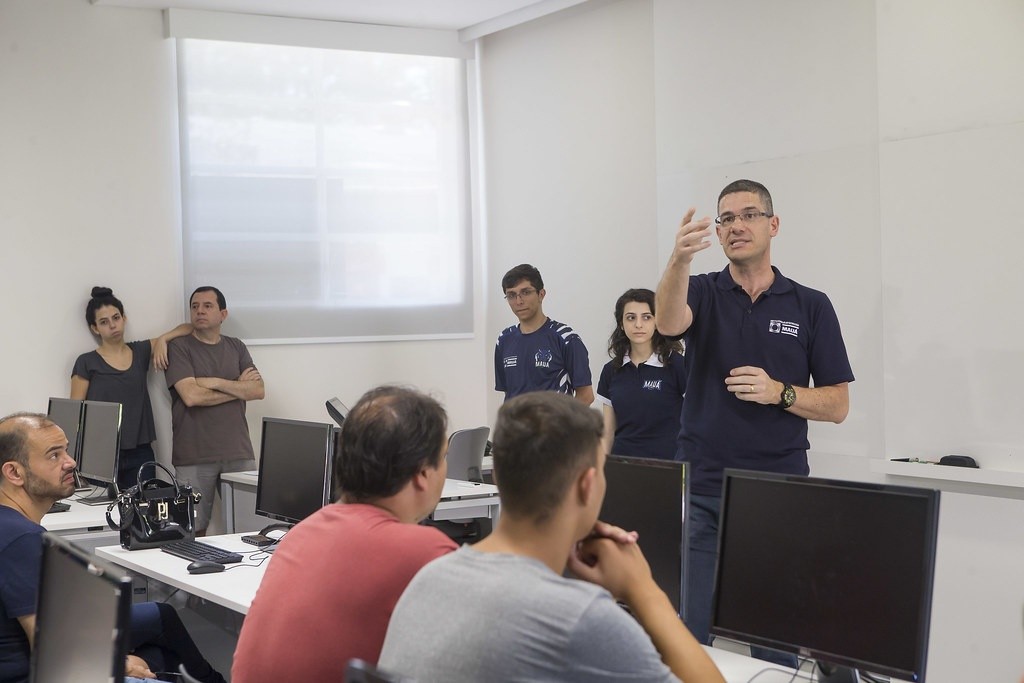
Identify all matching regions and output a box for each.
[187,560,225,574]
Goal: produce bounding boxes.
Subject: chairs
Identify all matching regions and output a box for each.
[444,426,491,539]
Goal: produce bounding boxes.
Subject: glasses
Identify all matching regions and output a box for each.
[504,287,542,302]
[714,210,773,224]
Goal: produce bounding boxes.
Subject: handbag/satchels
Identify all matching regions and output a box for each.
[105,461,202,552]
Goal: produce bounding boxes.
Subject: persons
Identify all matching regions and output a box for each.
[493,263,596,409]
[377,388,728,683]
[654,179,856,671]
[229,382,464,683]
[70,286,194,502]
[0,413,226,683]
[597,287,686,464]
[164,285,264,536]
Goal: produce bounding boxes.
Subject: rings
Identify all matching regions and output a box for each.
[750,385,754,393]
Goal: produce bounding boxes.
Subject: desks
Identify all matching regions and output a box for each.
[219,470,501,534]
[93,530,826,683]
[42,488,198,555]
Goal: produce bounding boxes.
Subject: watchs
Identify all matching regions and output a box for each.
[778,382,796,409]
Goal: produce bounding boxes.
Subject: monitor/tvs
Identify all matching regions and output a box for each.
[709,468,939,683]
[564,454,691,625]
[29,532,134,683]
[255,397,351,535]
[48,397,124,507]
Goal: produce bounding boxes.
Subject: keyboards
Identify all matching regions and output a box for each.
[160,541,245,564]
[46,502,71,514]
